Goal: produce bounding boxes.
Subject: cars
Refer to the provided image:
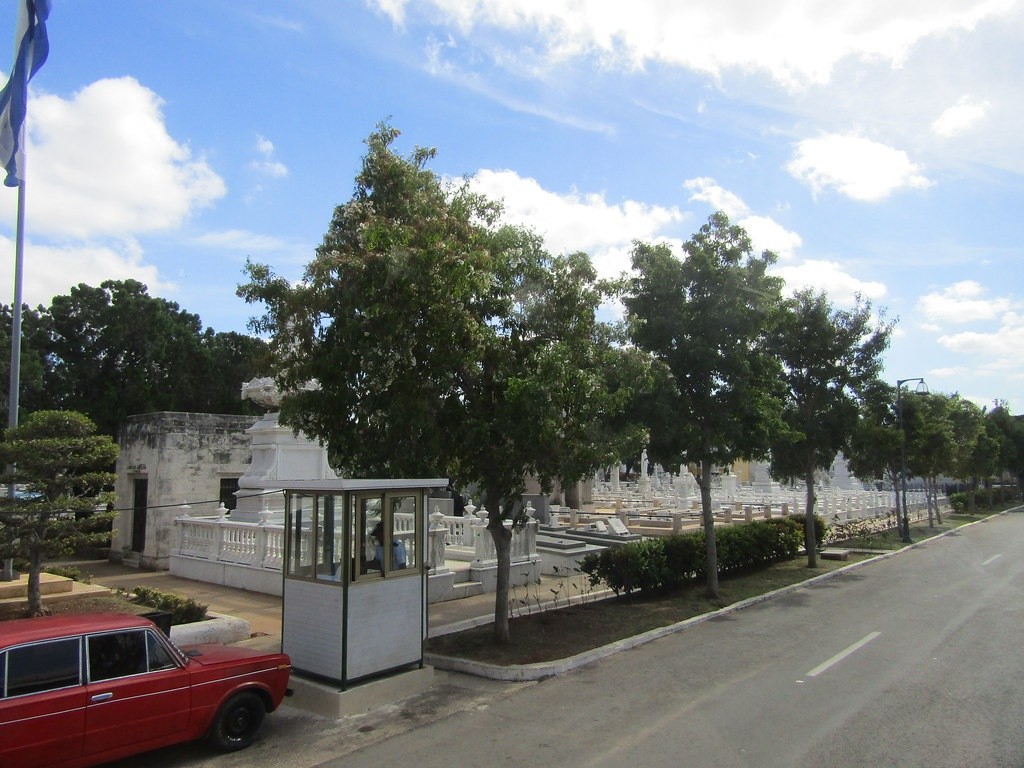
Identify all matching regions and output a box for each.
[1,614,290,767]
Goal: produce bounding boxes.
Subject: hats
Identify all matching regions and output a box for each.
[371,522,385,537]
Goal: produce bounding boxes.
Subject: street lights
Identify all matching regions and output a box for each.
[898,377,929,544]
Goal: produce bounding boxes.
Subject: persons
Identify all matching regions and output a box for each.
[366,522,407,578]
[641,449,649,475]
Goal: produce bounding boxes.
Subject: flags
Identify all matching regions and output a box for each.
[0,0,53,187]
